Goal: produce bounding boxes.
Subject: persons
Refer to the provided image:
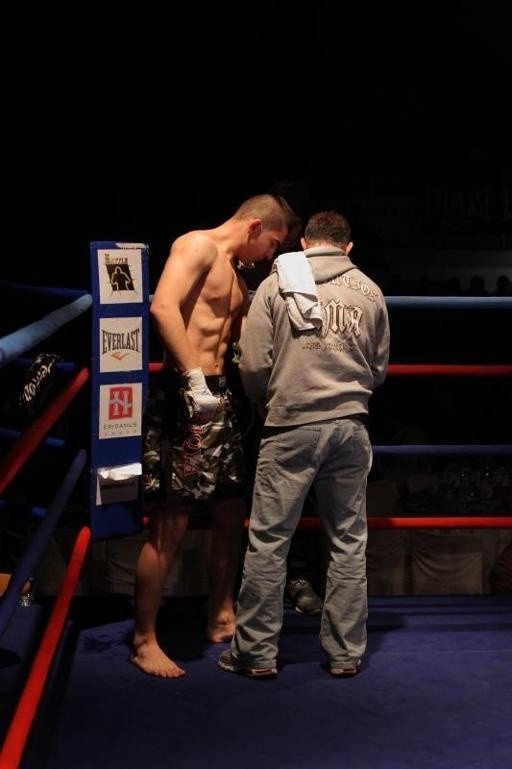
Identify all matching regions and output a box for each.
[128,192,293,680]
[216,211,390,680]
[109,531,512,610]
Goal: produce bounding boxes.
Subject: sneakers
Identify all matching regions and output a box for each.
[329,657,362,675]
[286,578,324,616]
[216,648,279,676]
